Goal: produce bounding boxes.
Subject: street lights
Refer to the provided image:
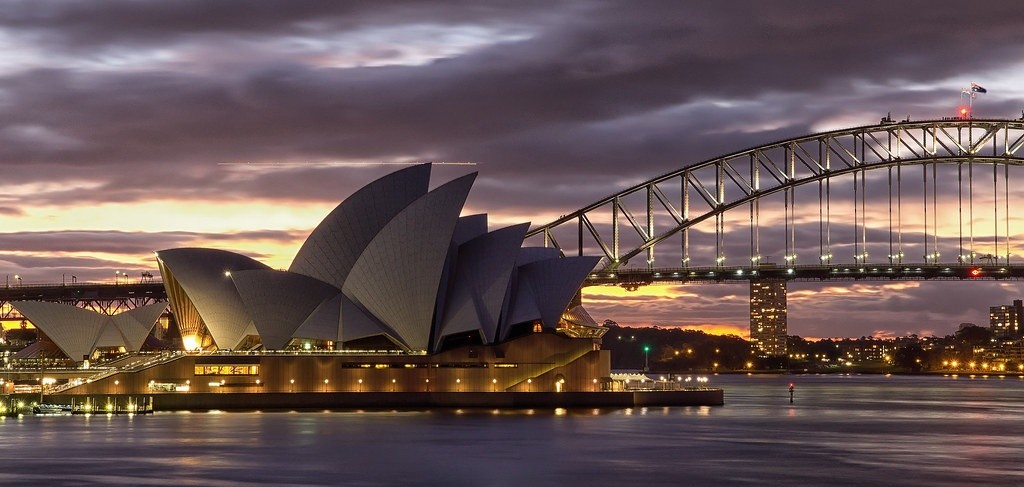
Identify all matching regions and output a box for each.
[645,347,649,367]
[114,380,119,395]
[392,379,396,392]
[116,271,120,285]
[359,379,362,393]
[325,379,328,392]
[14,274,22,287]
[257,380,260,392]
[493,378,497,392]
[290,379,294,392]
[122,273,128,284]
[528,379,531,392]
[426,378,429,392]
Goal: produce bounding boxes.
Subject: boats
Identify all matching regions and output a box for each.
[41,408,62,414]
